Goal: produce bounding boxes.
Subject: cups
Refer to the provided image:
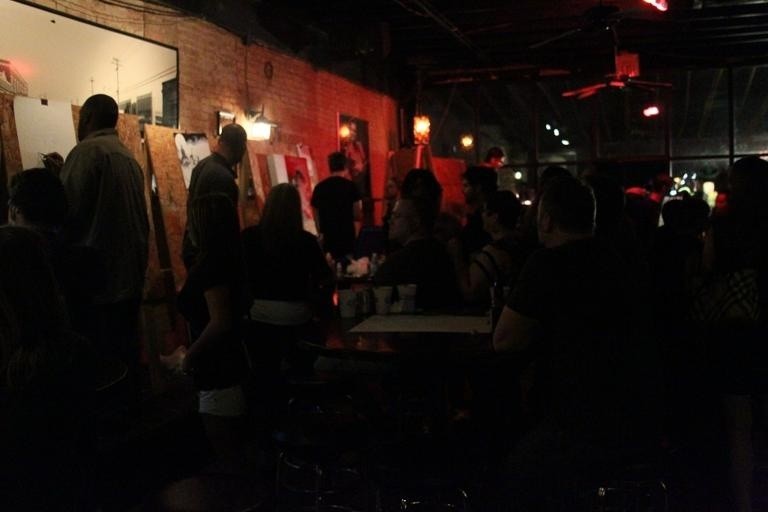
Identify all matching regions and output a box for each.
[373,286,392,315]
[398,284,416,313]
[338,289,356,319]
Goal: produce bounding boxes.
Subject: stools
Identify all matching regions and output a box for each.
[276,389,670,512]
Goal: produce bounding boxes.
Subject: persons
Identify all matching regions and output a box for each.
[2,89,767,510]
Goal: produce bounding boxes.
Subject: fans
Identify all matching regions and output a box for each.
[560,24,671,101]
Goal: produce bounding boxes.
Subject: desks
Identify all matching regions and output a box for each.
[350,314,493,334]
